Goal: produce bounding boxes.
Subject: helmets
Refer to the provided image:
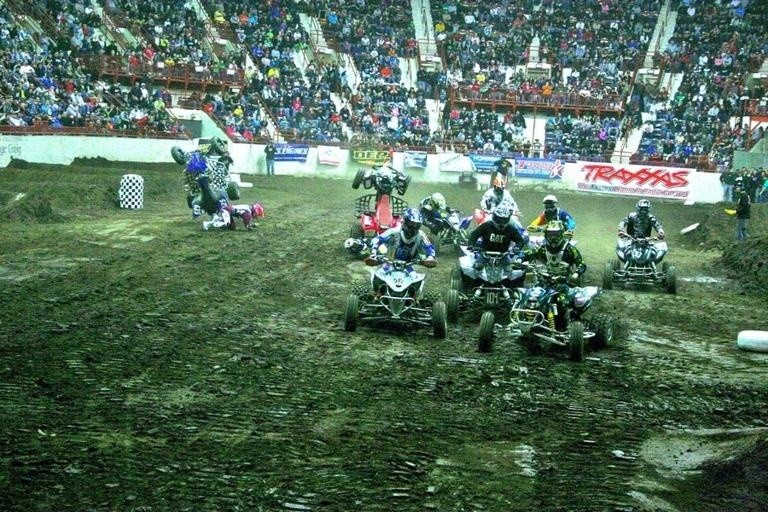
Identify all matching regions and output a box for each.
[493,173,505,192]
[344,238,361,251]
[541,194,557,212]
[401,207,423,235]
[543,221,563,247]
[429,191,448,211]
[635,199,652,218]
[248,201,263,218]
[493,201,514,224]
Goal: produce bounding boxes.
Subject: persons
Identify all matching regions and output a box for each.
[3,0,767,202]
[615,200,667,264]
[736,188,750,241]
[368,166,600,327]
[203,204,264,231]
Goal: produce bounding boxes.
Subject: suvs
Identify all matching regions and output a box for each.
[169,134,240,211]
